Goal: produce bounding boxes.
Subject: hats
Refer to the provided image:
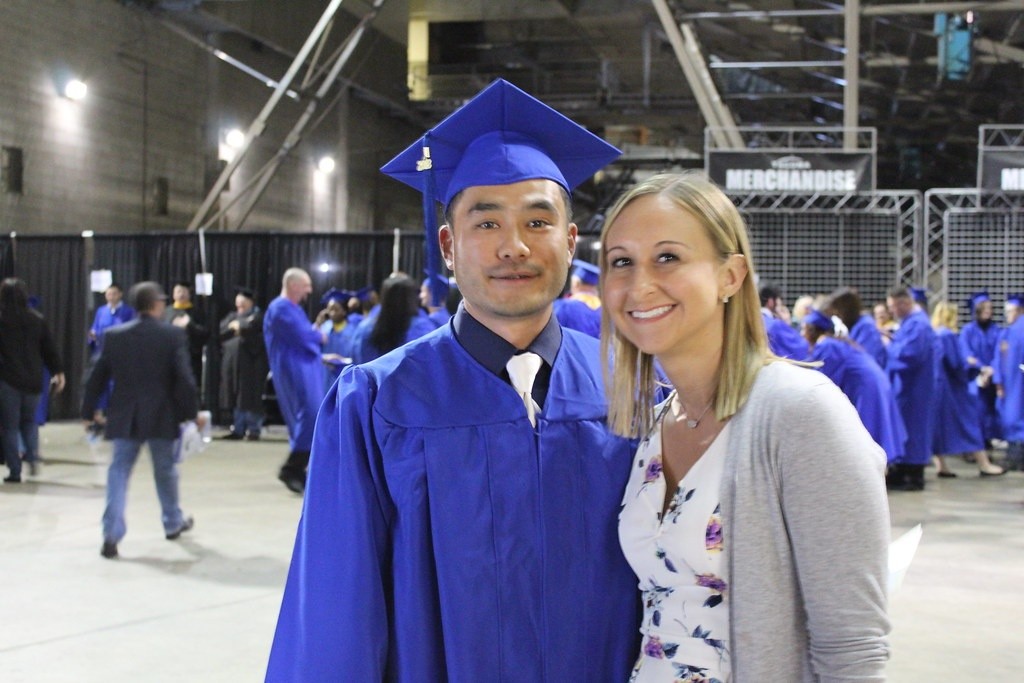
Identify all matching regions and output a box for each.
[909,288,928,305]
[802,308,834,330]
[380,75,623,308]
[970,292,989,314]
[321,287,355,304]
[1008,293,1024,306]
[423,268,451,299]
[359,285,372,300]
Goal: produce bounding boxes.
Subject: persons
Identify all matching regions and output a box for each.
[263,78,671,683]
[596,172,891,683]
[0,268,1022,558]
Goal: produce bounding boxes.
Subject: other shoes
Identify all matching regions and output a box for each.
[4,475,20,482]
[223,432,243,439]
[938,471,957,477]
[279,467,306,492]
[250,434,259,440]
[980,466,1006,475]
[101,541,117,559]
[165,517,193,540]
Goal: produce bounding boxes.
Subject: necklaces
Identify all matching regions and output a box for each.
[676,396,713,428]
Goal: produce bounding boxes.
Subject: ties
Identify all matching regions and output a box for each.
[505,350,541,427]
